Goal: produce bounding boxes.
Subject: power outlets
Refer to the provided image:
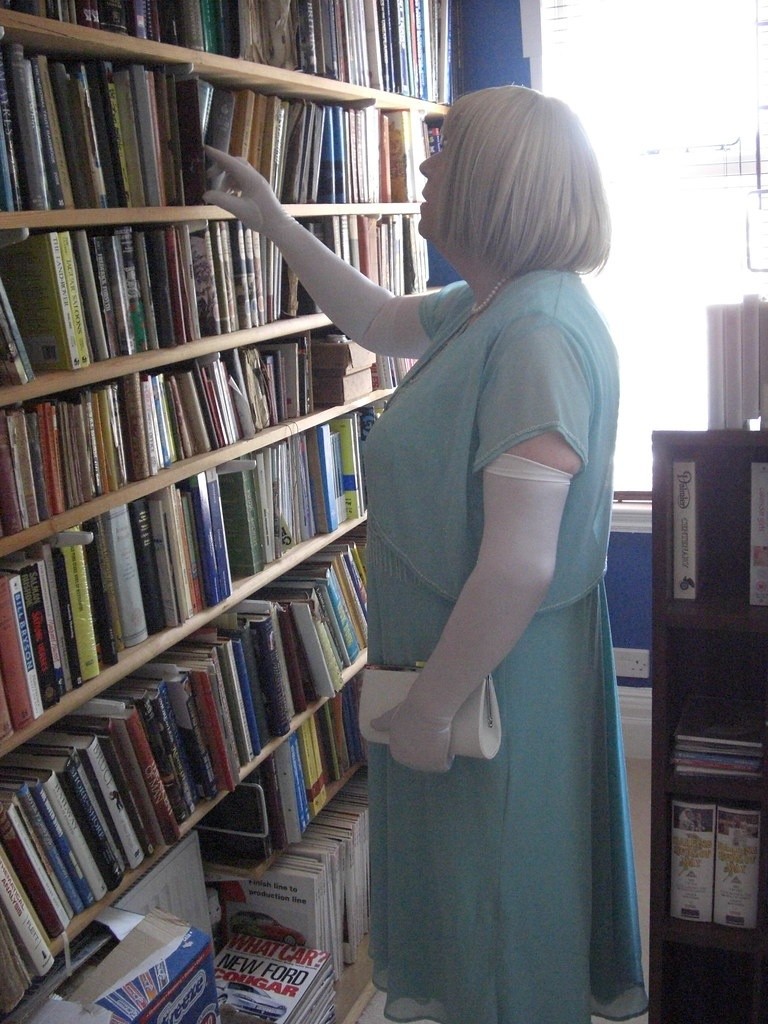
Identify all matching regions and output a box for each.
[614,648,650,678]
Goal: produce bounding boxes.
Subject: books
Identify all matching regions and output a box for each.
[672,457,767,607]
[668,686,768,930]
[710,295,767,430]
[0,0,449,1024]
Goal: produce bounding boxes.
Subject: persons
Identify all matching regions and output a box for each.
[201,86,648,1024]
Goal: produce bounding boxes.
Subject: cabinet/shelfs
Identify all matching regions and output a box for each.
[648,428,768,1024]
[0,7,448,1024]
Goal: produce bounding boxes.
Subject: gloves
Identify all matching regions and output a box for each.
[370,454,573,774]
[202,146,433,359]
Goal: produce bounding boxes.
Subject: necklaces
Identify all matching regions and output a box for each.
[470,274,509,317]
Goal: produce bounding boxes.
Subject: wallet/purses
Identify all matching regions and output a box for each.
[358,663,502,760]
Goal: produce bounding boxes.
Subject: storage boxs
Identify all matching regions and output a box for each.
[312,368,373,406]
[312,338,376,376]
[0,907,221,1024]
